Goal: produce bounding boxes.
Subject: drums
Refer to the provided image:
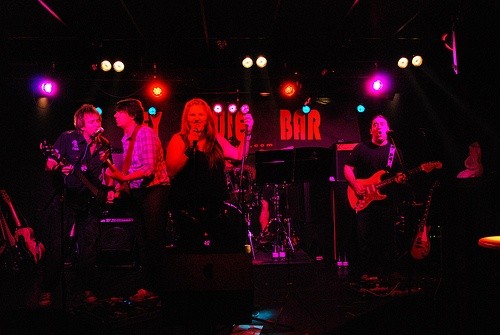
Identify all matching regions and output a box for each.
[224,169,254,205]
[223,202,246,217]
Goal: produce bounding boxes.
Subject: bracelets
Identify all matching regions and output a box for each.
[246,134,251,135]
[185,146,195,160]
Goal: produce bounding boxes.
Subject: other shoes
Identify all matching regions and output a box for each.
[38,293,53,307]
[79,290,97,303]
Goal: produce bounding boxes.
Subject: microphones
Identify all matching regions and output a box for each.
[94,127,104,137]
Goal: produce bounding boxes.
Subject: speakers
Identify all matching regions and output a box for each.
[325,293,500,335]
[158,252,253,321]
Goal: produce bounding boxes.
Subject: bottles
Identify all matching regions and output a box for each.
[271,240,286,261]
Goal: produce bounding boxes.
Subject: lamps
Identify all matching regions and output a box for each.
[390,51,408,69]
[404,49,423,67]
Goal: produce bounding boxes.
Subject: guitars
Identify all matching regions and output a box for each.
[0,188,45,264]
[409,181,439,260]
[40,139,107,213]
[347,160,443,213]
[96,126,129,202]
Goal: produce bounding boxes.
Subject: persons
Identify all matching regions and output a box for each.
[38,98,483,306]
[343,114,407,285]
